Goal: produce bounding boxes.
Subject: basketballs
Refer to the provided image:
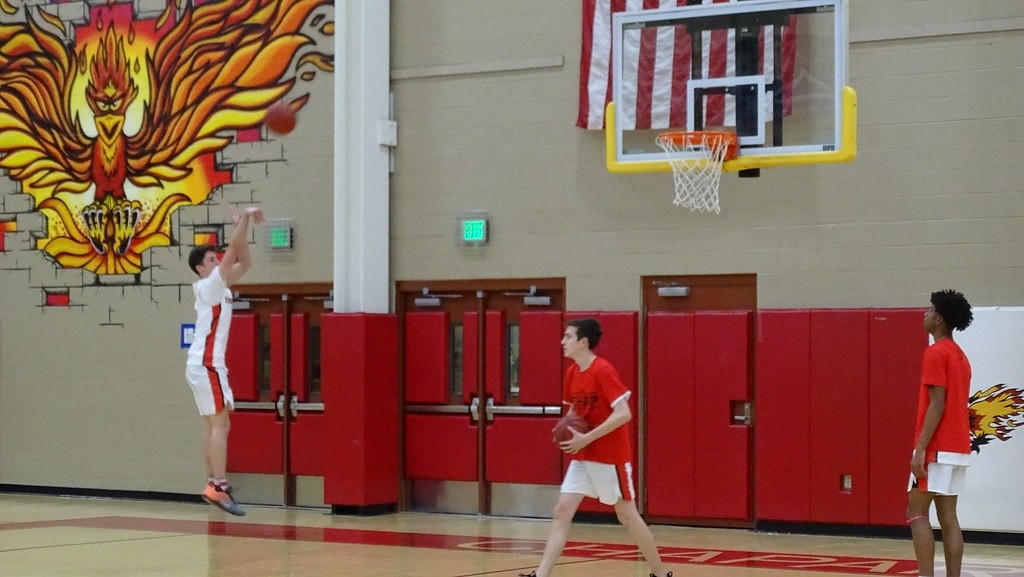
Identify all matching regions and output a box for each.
[555,414,587,451]
[266,103,297,134]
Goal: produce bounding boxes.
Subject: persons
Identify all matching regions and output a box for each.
[905,289,973,576]
[512,317,675,577]
[184,205,266,520]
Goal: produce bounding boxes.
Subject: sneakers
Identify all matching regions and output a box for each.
[203,483,246,516]
